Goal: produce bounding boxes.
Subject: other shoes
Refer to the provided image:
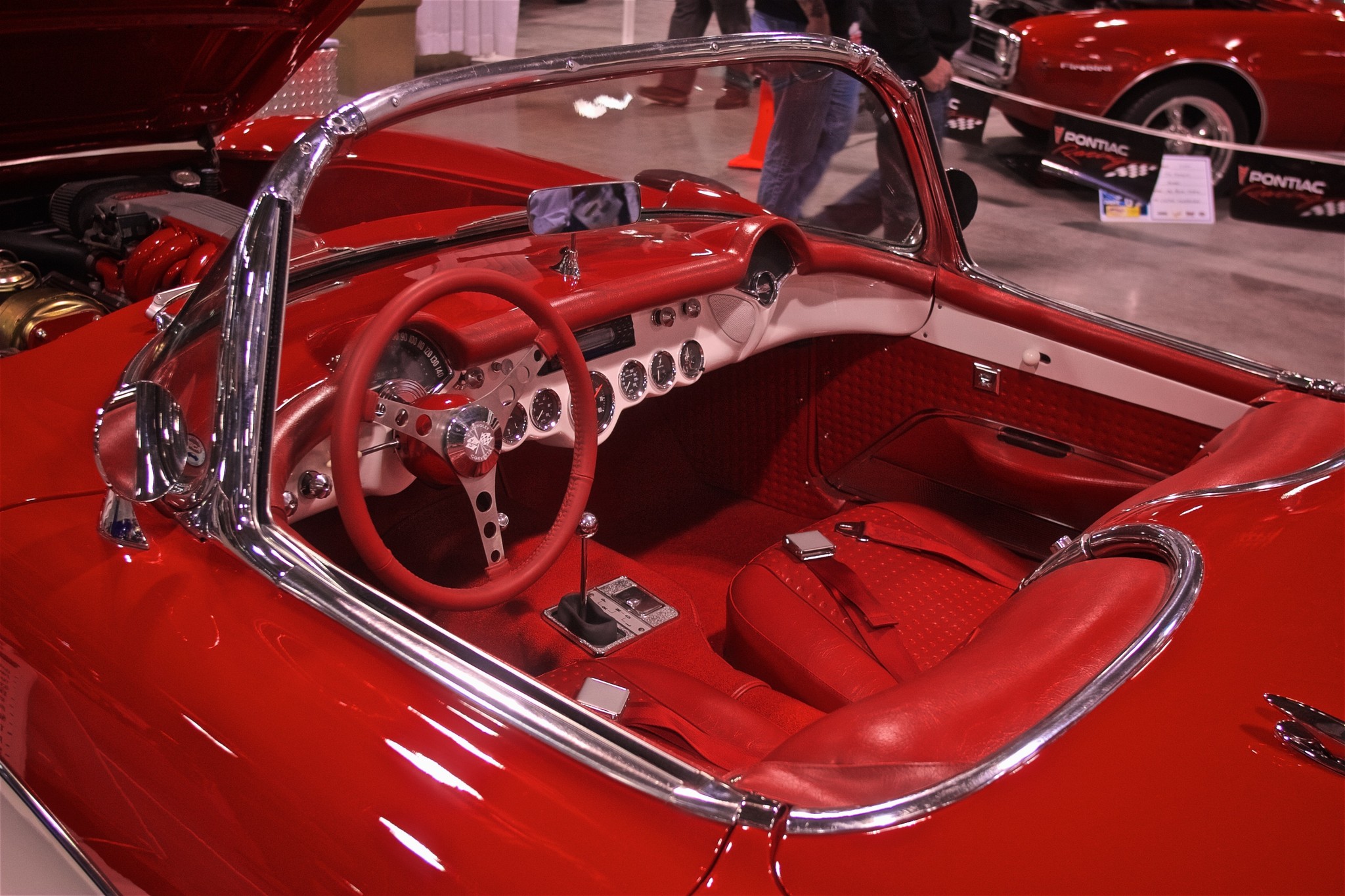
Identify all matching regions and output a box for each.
[715,90,751,108]
[636,83,686,104]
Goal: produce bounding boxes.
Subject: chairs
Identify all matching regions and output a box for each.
[528,557,1168,764]
[726,387,1345,714]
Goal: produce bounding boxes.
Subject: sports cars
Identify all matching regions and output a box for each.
[0,0,1345,896]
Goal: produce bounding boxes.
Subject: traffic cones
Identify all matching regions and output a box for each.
[728,76,777,173]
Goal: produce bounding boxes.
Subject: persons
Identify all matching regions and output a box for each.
[749,0,861,221]
[808,0,973,245]
[635,0,754,109]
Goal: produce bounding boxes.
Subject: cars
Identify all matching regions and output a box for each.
[963,0,1345,192]
